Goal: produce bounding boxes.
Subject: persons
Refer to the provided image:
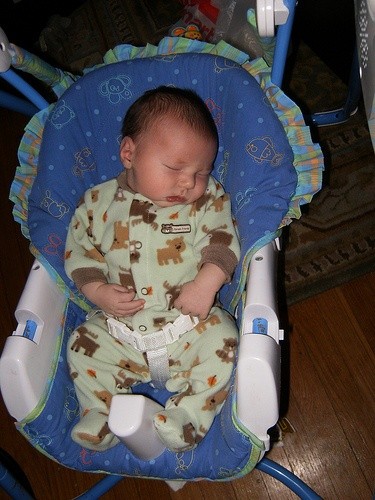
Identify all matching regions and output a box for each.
[67,86,242,451]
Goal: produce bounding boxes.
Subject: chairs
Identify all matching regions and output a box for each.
[1,1,322,499]
